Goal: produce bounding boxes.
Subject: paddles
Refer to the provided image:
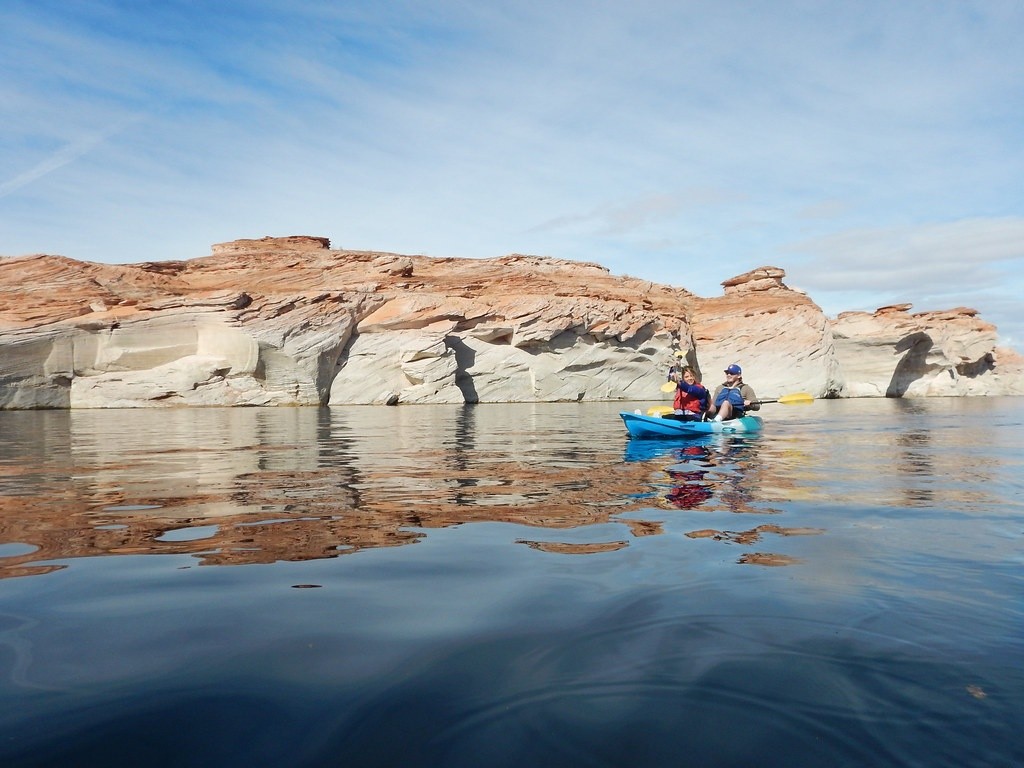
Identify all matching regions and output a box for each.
[647,392,814,420]
[660,350,689,394]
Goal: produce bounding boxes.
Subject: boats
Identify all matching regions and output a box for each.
[619,409,761,440]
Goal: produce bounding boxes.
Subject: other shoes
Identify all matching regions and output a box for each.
[707,417,722,422]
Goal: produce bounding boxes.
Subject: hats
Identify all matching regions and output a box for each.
[724,364,741,374]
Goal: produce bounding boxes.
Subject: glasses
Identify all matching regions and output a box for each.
[726,372,735,375]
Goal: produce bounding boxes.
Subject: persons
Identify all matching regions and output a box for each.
[634,367,716,421]
[707,364,760,421]
